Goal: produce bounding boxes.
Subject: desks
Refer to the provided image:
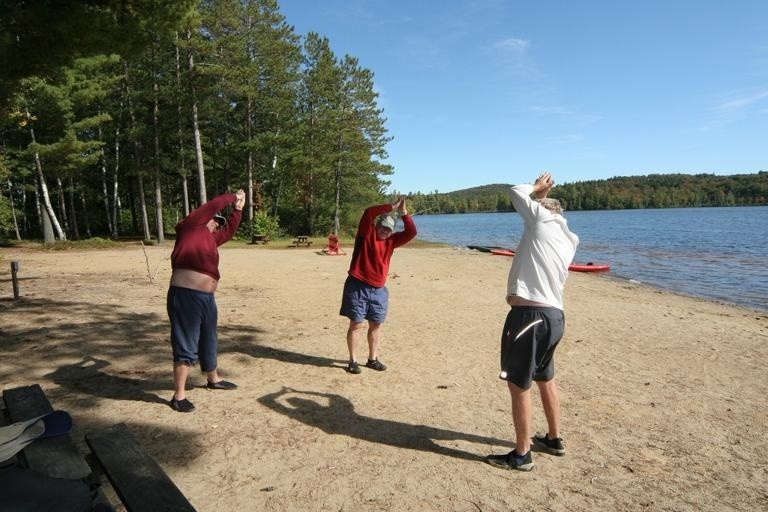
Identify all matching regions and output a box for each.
[1,382,118,512]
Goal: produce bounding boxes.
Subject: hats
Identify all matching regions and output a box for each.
[213,213,226,228]
[378,215,397,232]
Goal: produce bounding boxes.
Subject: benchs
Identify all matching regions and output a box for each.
[84,423,203,512]
[251,233,313,247]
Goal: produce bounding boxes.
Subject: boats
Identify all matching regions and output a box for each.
[488,248,610,273]
[466,243,504,252]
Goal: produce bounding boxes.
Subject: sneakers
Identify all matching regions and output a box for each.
[533,433,565,456]
[348,362,360,374]
[487,448,534,471]
[206,378,237,389]
[170,397,196,412]
[366,357,386,370]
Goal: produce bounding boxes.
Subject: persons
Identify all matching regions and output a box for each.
[165,190,246,411]
[486,172,579,473]
[339,196,418,374]
